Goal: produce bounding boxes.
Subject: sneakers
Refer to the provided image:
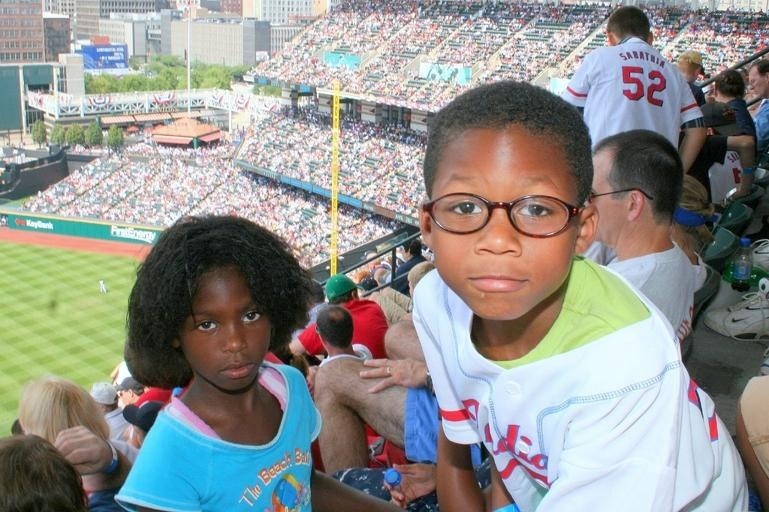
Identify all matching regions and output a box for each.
[752,239,769,271]
[705,278,768,340]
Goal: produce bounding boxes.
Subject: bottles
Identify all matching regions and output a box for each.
[731,236,754,293]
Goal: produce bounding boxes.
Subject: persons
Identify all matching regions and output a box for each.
[413,81,749,512]
[638,3,769,85]
[672,50,769,291]
[113,216,323,511]
[314,129,695,512]
[560,5,706,173]
[23,103,429,268]
[251,0,621,111]
[0,359,181,512]
[266,237,436,467]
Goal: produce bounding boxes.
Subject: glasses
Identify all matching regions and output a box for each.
[586,188,653,204]
[424,192,582,238]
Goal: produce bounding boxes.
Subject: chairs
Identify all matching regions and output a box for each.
[678,167,769,357]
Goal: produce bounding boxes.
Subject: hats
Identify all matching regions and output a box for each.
[679,50,705,75]
[91,377,165,432]
[700,102,736,127]
[326,274,362,299]
